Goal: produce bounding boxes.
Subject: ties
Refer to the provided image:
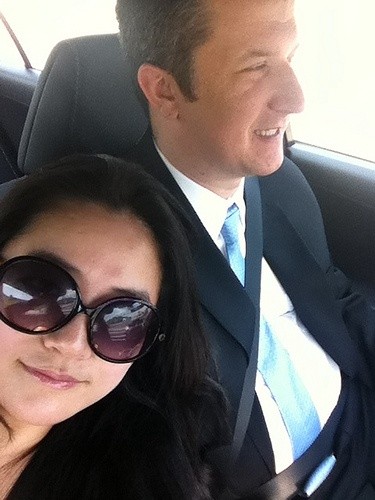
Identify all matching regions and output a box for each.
[222,203,338,497]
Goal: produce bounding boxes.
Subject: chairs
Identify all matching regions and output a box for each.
[0,34,150,207]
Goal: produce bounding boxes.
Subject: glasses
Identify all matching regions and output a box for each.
[0,254,168,365]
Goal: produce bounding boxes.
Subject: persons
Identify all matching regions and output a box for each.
[113,1,374,500]
[0,154,231,500]
